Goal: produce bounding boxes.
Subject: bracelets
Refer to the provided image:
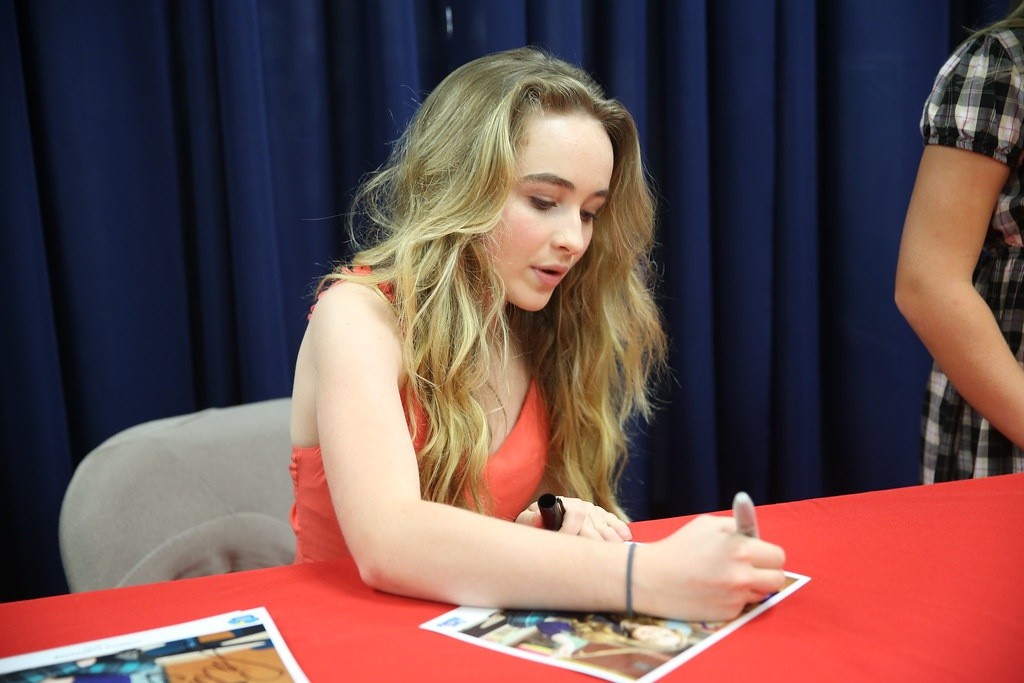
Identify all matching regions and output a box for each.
[625,544,637,617]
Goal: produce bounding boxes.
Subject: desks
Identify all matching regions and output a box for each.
[1,471,1024,683]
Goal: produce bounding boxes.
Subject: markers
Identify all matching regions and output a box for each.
[733,492,757,538]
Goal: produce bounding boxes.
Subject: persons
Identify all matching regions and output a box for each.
[286,46,787,623]
[894,0,1024,490]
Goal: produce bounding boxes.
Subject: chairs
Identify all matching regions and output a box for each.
[57,396,298,593]
